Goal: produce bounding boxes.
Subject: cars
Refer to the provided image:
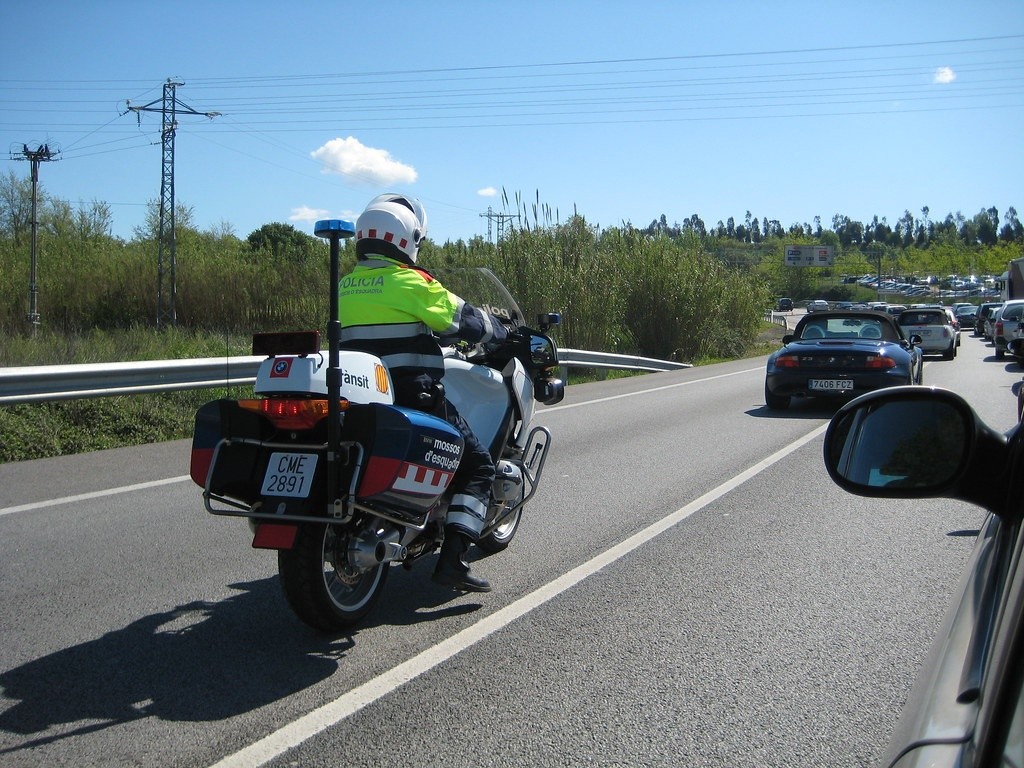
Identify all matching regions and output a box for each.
[841,272,1002,299]
[806,300,830,313]
[956,306,980,328]
[981,306,1001,342]
[911,307,962,348]
[896,307,957,361]
[833,301,973,322]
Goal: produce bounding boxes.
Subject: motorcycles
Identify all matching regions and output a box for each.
[191,218,569,636]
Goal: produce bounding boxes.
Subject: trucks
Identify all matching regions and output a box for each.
[997,257,1024,303]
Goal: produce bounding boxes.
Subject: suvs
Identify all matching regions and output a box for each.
[776,297,794,312]
[990,300,1024,359]
[970,303,1004,338]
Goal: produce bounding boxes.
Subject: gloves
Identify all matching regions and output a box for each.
[503,324,517,337]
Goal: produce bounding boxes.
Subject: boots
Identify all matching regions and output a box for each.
[432,531,492,592]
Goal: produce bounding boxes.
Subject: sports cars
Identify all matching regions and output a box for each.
[765,310,924,413]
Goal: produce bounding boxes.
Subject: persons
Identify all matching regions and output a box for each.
[339,193,518,592]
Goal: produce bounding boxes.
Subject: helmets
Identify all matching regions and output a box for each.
[355,202,422,265]
